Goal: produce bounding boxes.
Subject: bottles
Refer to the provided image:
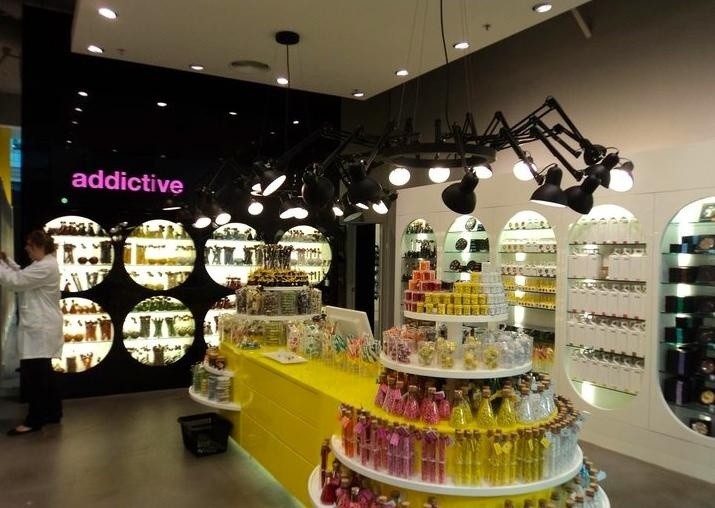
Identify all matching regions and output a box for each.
[40,215,328,375]
[321,259,606,508]
[564,216,652,401]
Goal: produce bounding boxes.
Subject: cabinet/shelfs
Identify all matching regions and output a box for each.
[41,217,328,415]
[309,197,713,507]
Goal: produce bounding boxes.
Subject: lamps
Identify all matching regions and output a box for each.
[161,94,638,229]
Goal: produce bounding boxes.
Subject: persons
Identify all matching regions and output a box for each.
[0,228,64,436]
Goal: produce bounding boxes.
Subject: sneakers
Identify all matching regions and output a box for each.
[6,418,61,435]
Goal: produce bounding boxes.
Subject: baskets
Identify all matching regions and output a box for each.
[177,412,233,457]
